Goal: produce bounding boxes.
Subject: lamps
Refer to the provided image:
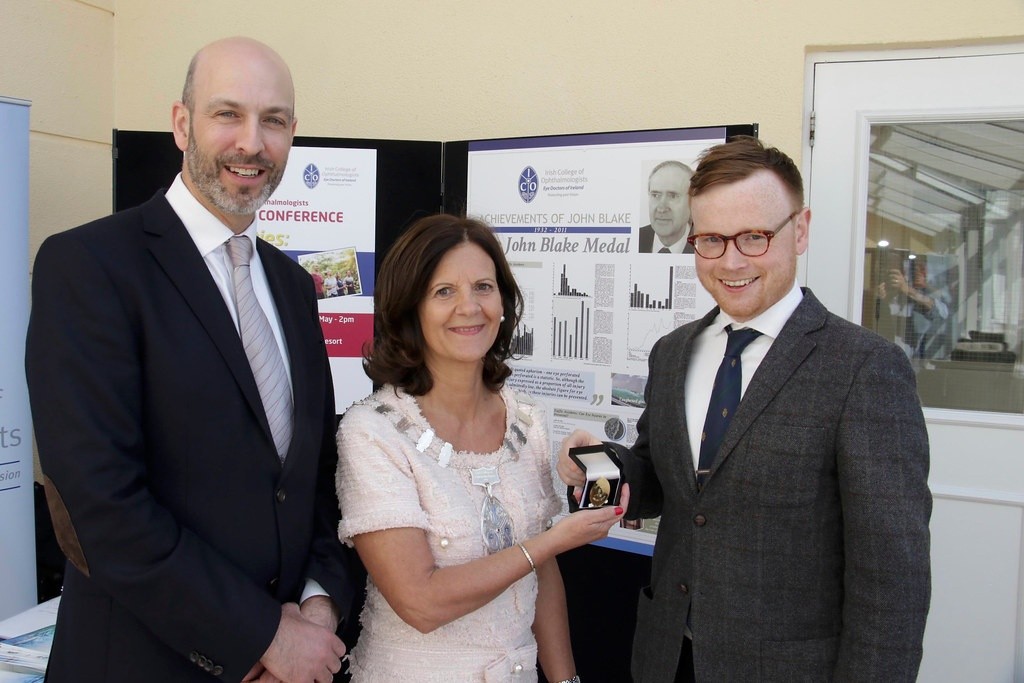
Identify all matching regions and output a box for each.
[907,250,918,261]
[877,217,889,247]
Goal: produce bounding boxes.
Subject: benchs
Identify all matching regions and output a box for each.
[950,339,1016,363]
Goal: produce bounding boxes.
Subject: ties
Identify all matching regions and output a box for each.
[658,248,670,253]
[226,237,294,466]
[687,324,764,634]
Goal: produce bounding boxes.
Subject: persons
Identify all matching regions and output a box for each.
[873,254,953,357]
[639,161,696,253]
[335,214,632,683]
[558,138,933,683]
[24,38,346,683]
[312,264,356,299]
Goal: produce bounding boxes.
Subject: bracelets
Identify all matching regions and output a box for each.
[518,543,536,571]
[558,676,580,683]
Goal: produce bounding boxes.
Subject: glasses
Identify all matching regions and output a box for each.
[687,212,798,259]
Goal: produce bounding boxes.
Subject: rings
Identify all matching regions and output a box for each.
[896,280,898,284]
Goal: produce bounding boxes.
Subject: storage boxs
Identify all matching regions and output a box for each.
[569,445,626,511]
